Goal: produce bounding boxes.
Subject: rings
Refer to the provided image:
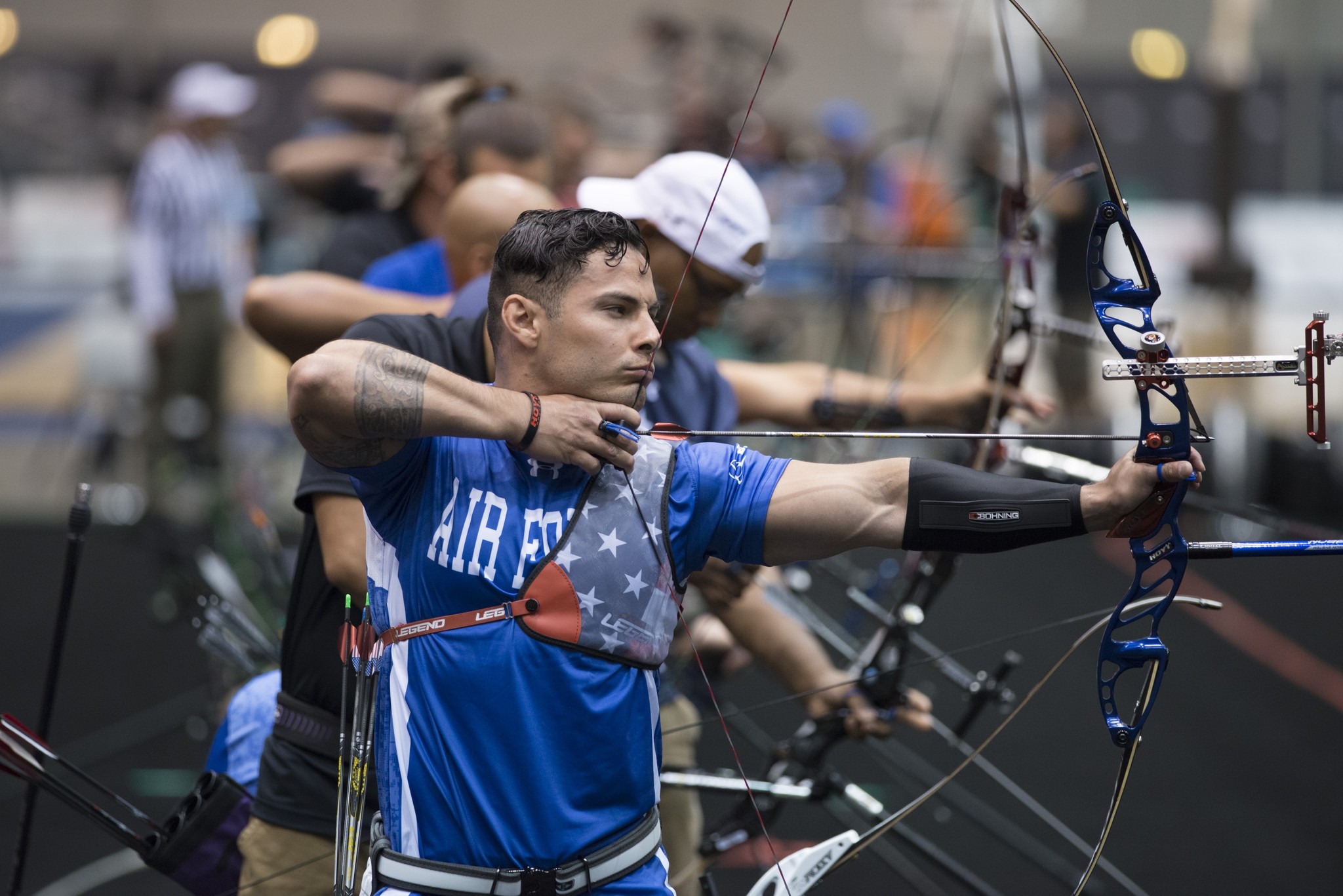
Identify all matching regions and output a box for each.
[1157,462,1167,483]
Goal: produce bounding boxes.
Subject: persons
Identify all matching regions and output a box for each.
[206,11,1207,896]
[131,59,261,556]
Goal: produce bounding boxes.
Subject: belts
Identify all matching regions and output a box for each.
[373,805,663,896]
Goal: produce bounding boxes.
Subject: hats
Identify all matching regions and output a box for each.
[576,149,772,286]
[166,61,257,124]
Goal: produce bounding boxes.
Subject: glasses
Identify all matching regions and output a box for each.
[681,251,734,308]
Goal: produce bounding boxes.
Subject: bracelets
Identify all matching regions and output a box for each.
[507,391,541,452]
